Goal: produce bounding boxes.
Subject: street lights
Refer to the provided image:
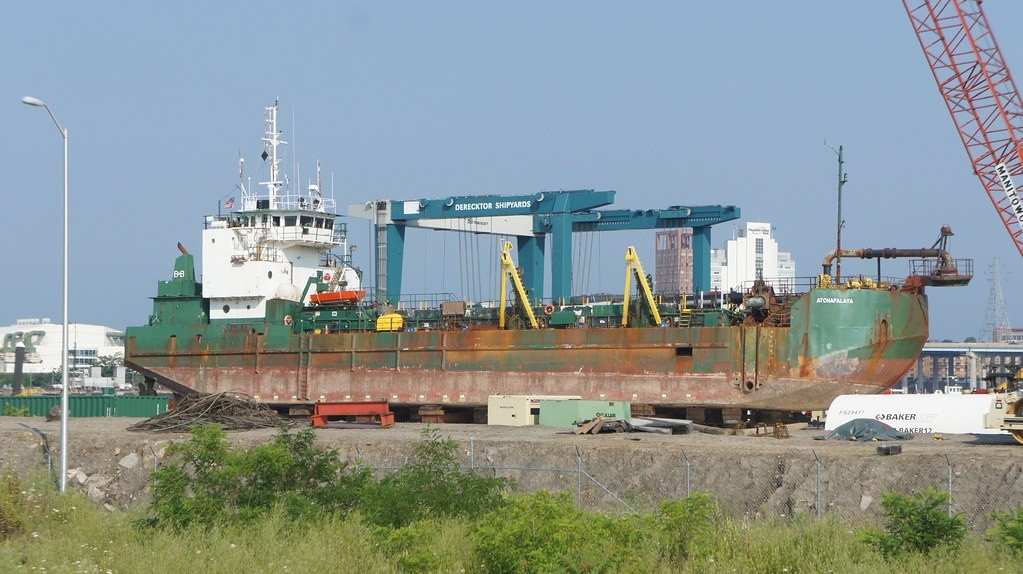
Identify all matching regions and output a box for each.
[21,95,69,493]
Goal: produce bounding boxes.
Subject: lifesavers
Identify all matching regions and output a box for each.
[544,304,555,315]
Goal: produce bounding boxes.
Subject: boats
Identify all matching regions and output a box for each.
[124,96,973,409]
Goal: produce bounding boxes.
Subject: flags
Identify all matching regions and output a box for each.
[224,197,234,208]
